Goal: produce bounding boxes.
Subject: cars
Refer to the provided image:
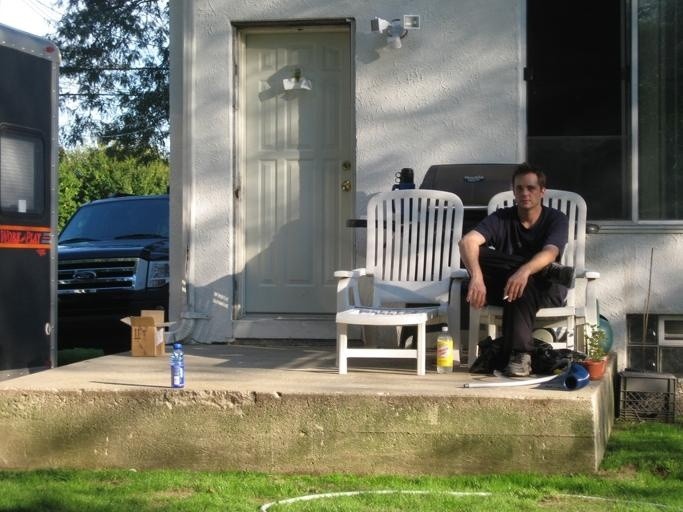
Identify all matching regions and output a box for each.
[57,196,169,329]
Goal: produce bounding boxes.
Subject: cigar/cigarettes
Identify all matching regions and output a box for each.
[502,295,509,301]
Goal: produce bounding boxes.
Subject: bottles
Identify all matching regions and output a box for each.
[169,342,184,387]
[435,325,455,373]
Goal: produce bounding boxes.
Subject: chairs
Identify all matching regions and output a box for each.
[333,188,460,375]
[450,189,587,368]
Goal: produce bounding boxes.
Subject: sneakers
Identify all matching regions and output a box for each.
[505,351,533,379]
[546,262,577,290]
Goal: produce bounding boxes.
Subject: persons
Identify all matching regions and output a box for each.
[458,164,575,377]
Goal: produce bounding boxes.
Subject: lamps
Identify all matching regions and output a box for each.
[368,12,420,48]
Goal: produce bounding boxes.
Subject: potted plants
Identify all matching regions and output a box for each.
[579,329,609,381]
[598,326,609,370]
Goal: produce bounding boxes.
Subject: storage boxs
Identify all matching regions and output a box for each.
[120,310,175,357]
